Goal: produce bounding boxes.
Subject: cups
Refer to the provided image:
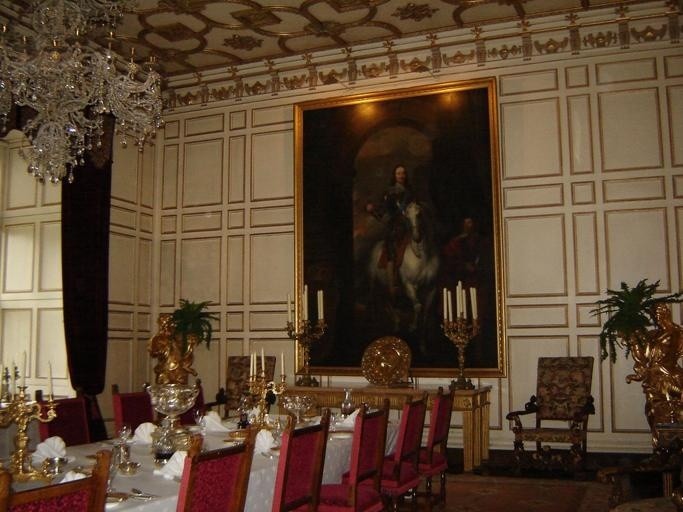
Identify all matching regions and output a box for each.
[330,412,340,430]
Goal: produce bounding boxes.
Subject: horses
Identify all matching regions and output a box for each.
[369,197,443,335]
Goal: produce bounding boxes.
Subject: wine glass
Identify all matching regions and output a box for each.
[234,393,314,432]
[147,383,201,449]
[39,420,138,507]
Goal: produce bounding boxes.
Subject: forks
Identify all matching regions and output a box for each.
[127,488,159,502]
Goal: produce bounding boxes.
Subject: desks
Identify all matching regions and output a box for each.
[278,384,492,474]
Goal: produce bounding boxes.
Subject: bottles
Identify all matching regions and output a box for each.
[341,388,355,420]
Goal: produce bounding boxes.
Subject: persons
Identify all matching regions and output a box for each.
[448,218,494,318]
[352,162,415,289]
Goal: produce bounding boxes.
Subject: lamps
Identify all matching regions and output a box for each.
[0,0,166,184]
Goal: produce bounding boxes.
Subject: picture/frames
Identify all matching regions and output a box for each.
[293,74,508,379]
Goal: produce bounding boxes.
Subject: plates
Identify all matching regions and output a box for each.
[361,335,412,386]
[229,431,253,439]
[1,400,36,409]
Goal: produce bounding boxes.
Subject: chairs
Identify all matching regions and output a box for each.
[205,356,277,420]
[176,415,265,512]
[0,450,111,512]
[111,381,154,439]
[315,399,391,512]
[177,378,207,426]
[594,393,683,511]
[341,390,423,511]
[33,389,92,448]
[385,379,456,511]
[272,408,331,512]
[506,356,596,480]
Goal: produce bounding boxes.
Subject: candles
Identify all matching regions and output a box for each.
[317,291,320,320]
[249,347,285,377]
[443,280,478,322]
[304,285,308,320]
[321,290,324,320]
[287,294,291,323]
